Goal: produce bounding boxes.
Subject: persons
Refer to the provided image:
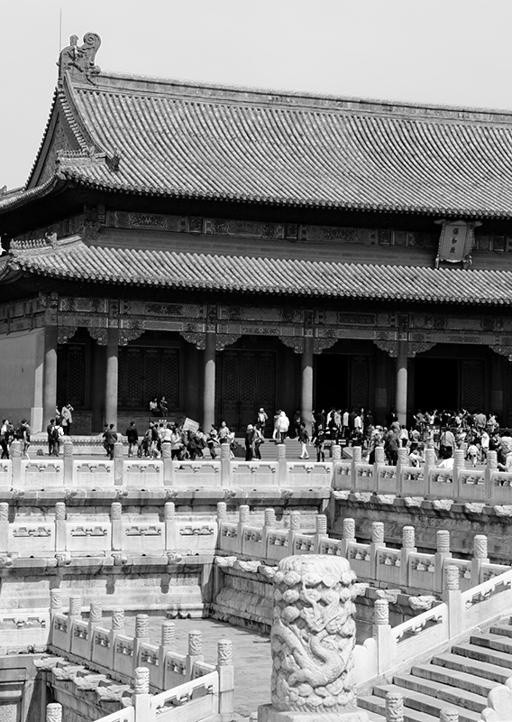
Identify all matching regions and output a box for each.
[126,395,236,460]
[244,407,511,486]
[102,424,118,460]
[0,417,31,460]
[47,402,74,456]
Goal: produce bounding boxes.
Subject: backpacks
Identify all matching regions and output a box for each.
[53,426,60,439]
